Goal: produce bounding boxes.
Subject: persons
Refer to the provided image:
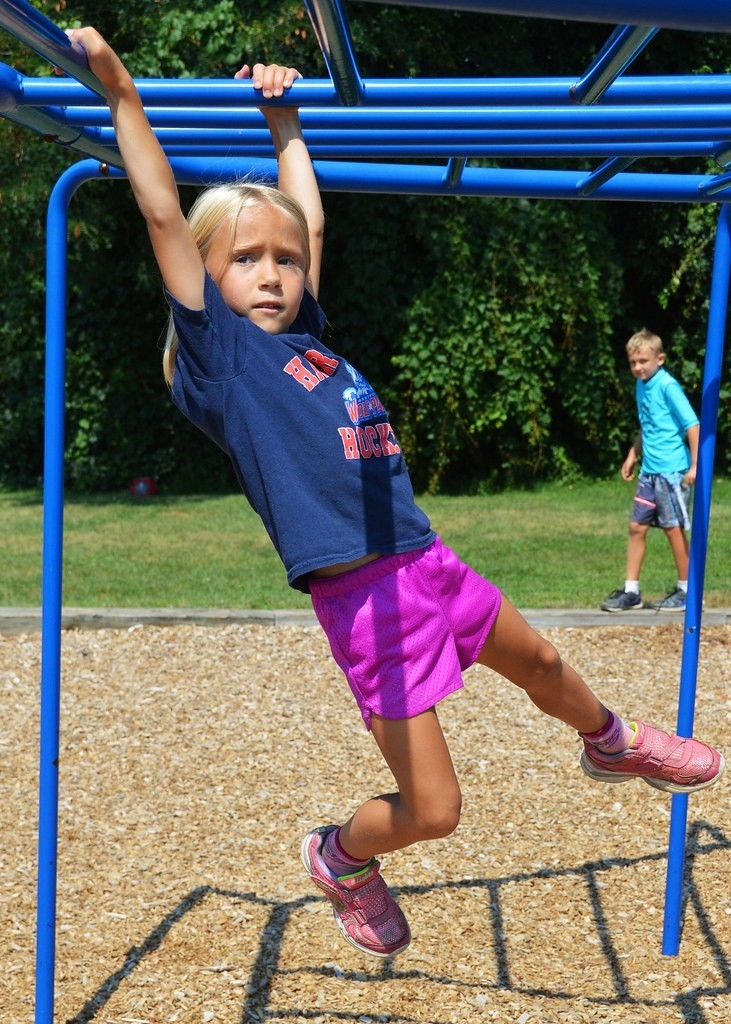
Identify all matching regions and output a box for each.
[600,328,702,615]
[55,22,727,956]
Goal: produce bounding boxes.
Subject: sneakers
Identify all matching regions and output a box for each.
[299,825,412,958]
[650,587,705,612]
[579,721,727,794]
[601,586,644,613]
